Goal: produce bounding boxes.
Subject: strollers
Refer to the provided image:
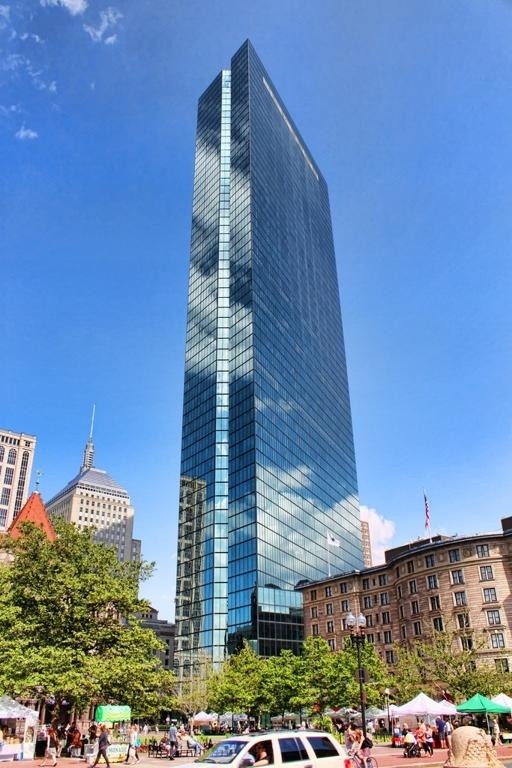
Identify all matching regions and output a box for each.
[404,733,421,758]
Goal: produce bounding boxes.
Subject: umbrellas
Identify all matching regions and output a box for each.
[187,689,399,735]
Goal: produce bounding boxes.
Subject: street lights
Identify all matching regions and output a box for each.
[385,688,392,733]
[345,611,373,748]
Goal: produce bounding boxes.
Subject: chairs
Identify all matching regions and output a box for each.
[148,738,206,759]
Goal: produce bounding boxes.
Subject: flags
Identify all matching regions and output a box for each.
[423,494,431,528]
[327,534,341,548]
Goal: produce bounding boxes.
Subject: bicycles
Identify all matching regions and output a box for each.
[349,751,378,768]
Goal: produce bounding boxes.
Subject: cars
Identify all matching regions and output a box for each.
[168,729,351,768]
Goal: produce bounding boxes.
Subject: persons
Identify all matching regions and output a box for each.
[349,728,373,768]
[37,720,211,768]
[25,725,37,743]
[344,722,355,751]
[251,742,272,766]
[390,714,504,758]
